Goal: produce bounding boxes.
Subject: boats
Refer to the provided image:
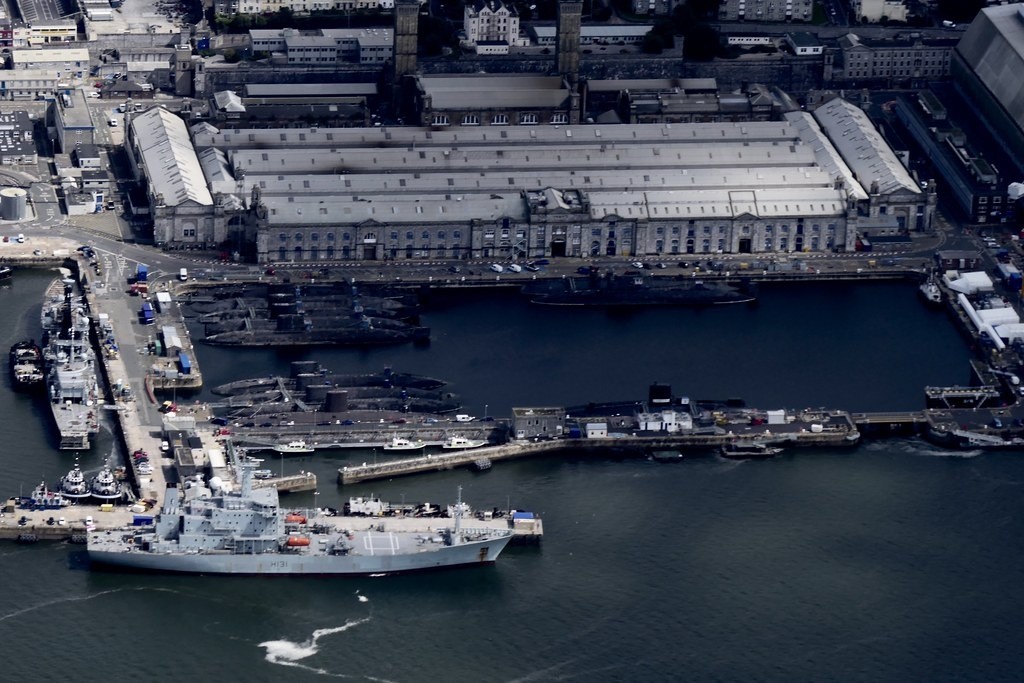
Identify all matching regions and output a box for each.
[443,434,486,448]
[383,436,428,450]
[919,267,942,303]
[10,266,105,449]
[271,442,315,453]
[722,439,783,456]
[18,452,136,504]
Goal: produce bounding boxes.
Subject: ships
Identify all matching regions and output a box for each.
[211,362,463,422]
[566,383,745,416]
[198,284,429,346]
[87,447,515,574]
